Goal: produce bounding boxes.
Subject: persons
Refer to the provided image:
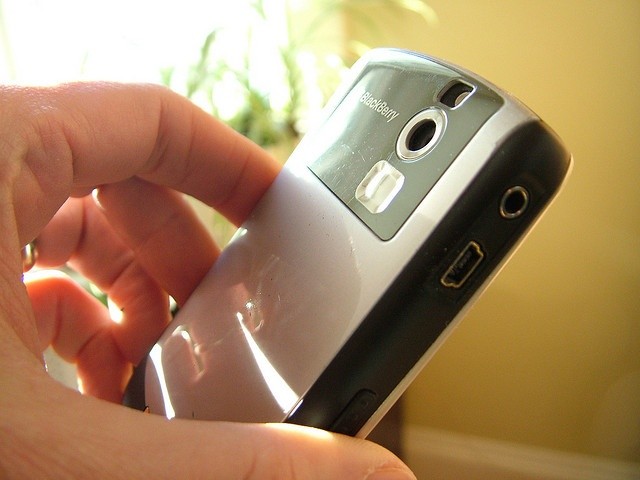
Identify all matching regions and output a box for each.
[0,80,417,480]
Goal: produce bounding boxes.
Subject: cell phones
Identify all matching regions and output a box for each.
[119,45,573,441]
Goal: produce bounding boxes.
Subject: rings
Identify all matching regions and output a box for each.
[24,239,38,272]
